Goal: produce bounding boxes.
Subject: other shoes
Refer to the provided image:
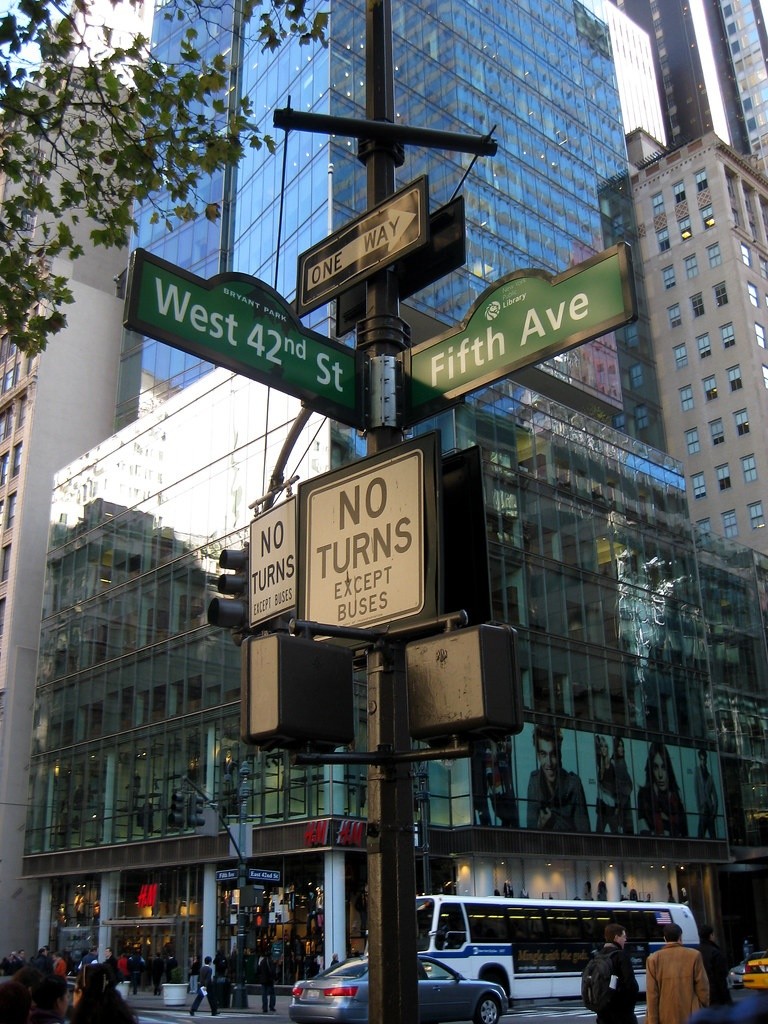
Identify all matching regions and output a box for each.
[212,1013,217,1016]
[189,1011,194,1016]
[263,1009,268,1012]
[270,1007,276,1011]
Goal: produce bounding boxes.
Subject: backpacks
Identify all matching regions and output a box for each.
[581,949,622,1011]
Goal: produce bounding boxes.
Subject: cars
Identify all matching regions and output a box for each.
[288,955,509,1024]
[726,957,749,990]
[0,975,198,1024]
[743,949,768,991]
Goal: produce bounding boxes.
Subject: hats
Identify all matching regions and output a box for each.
[11,951,16,955]
[698,926,714,938]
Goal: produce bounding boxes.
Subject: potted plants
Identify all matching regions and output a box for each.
[162,967,189,1006]
[115,971,131,1000]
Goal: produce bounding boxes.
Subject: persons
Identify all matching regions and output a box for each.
[590,923,640,1024]
[291,935,306,984]
[270,935,285,984]
[694,924,732,1008]
[471,744,519,829]
[637,742,687,838]
[526,723,591,834]
[694,750,719,840]
[259,951,277,1012]
[645,923,710,1024]
[593,734,634,836]
[331,953,339,967]
[0,944,179,1024]
[493,878,691,909]
[188,949,238,1016]
[57,889,100,928]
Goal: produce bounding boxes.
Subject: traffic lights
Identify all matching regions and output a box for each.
[254,914,267,927]
[208,547,249,634]
[187,793,205,826]
[167,790,185,827]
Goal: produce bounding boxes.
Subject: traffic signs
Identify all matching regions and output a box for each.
[294,172,429,319]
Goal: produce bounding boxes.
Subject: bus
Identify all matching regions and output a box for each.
[361,893,700,1007]
